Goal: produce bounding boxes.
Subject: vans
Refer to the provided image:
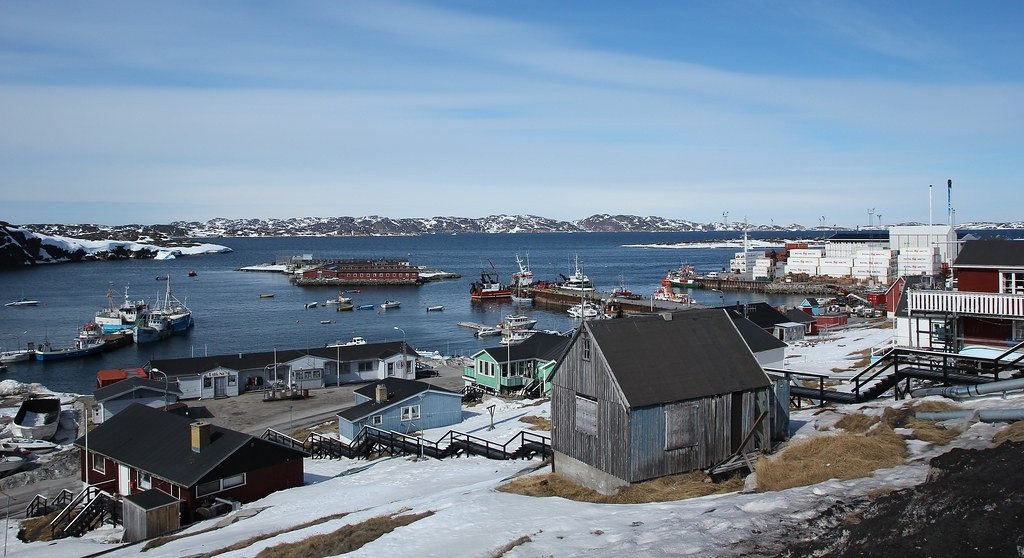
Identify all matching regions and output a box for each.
[706,271,719,279]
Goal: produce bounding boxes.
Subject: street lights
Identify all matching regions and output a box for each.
[711,288,725,306]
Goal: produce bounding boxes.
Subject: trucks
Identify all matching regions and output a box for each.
[857,308,874,318]
[873,311,882,317]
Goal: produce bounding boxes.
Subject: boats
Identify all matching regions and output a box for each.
[603,270,642,300]
[344,289,361,294]
[0,454,27,472]
[189,271,197,276]
[337,304,354,311]
[304,301,318,308]
[498,328,561,347]
[260,294,274,298]
[555,252,597,291]
[4,300,41,307]
[566,300,602,318]
[474,314,538,337]
[10,397,61,441]
[662,259,704,288]
[0,348,38,363]
[427,305,444,311]
[510,250,533,286]
[510,282,533,304]
[133,306,175,344]
[75,273,192,347]
[326,297,353,304]
[469,256,511,298]
[155,276,170,281]
[356,304,375,310]
[381,300,401,309]
[0,438,59,455]
[458,321,493,330]
[651,279,697,305]
[595,293,623,319]
[33,329,105,363]
